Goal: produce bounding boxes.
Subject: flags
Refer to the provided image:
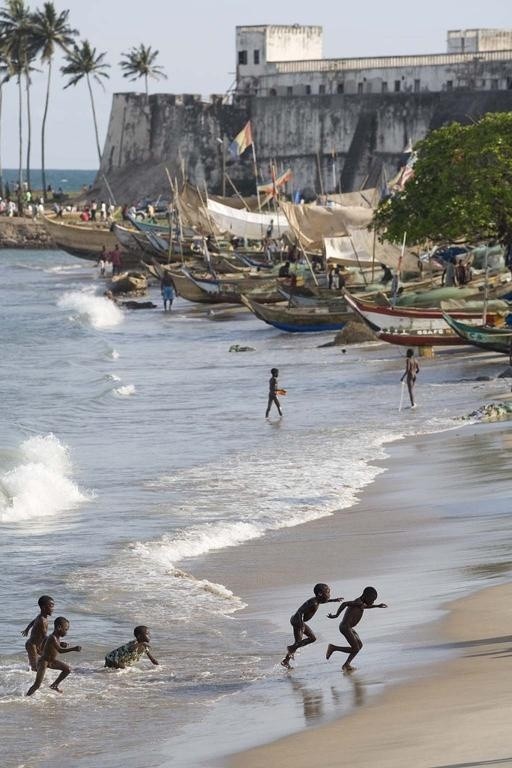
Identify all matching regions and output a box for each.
[226,120,258,161]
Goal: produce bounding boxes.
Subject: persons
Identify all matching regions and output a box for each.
[94,241,124,278]
[333,268,346,290]
[440,255,458,288]
[104,625,159,671]
[399,349,419,408]
[280,263,290,276]
[28,617,82,697]
[462,260,474,284]
[325,585,388,672]
[281,583,344,669]
[18,595,56,671]
[161,270,179,312]
[0,181,158,224]
[266,219,274,249]
[454,259,467,287]
[342,348,346,353]
[379,263,393,281]
[264,368,288,418]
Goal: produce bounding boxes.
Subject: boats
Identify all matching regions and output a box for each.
[42,120,450,332]
[342,232,511,348]
[441,267,512,352]
